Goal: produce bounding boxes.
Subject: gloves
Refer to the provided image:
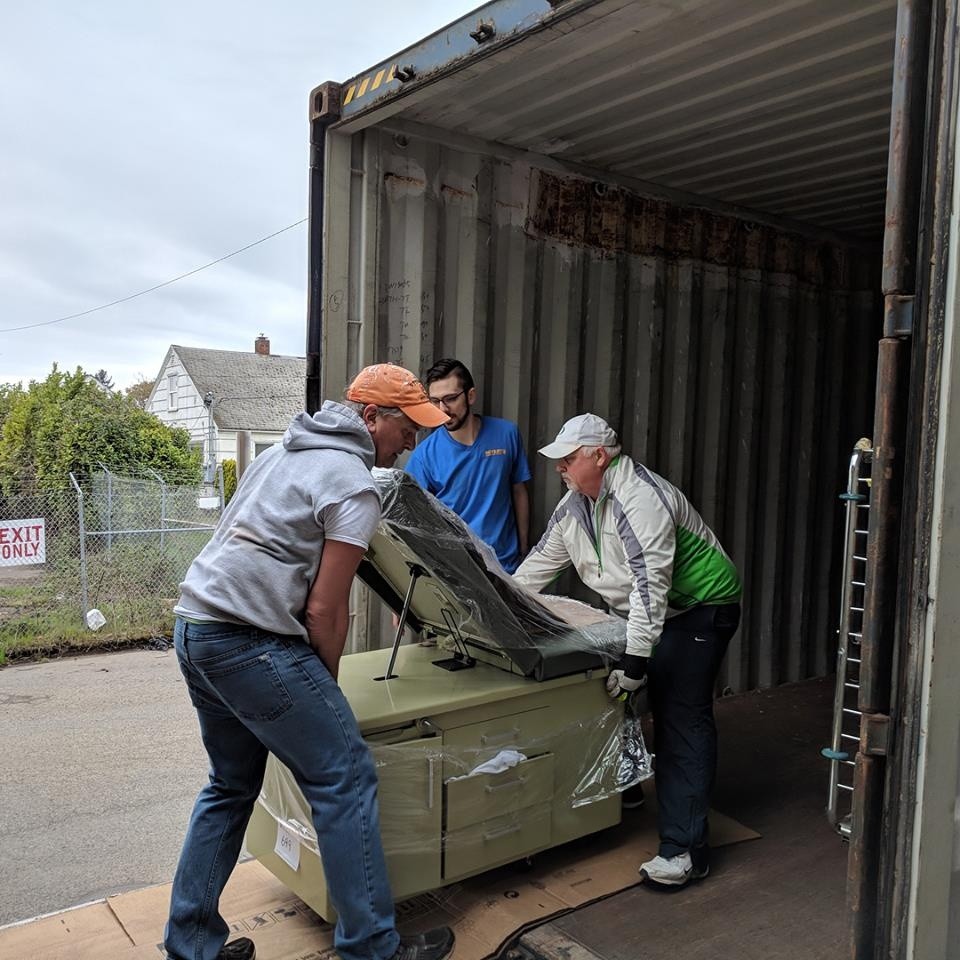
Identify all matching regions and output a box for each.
[605,668,649,702]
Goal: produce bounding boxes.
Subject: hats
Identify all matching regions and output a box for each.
[346,362,452,428]
[537,413,618,460]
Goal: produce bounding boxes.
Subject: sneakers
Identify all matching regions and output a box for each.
[390,926,457,960]
[637,852,708,886]
[623,789,646,807]
[218,935,255,960]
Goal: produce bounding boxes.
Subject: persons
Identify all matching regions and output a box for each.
[163,362,458,960]
[511,412,743,890]
[392,358,531,635]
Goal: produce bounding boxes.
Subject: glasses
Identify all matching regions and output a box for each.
[431,387,471,407]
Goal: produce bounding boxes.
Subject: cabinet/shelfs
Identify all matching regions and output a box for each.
[243,463,627,927]
